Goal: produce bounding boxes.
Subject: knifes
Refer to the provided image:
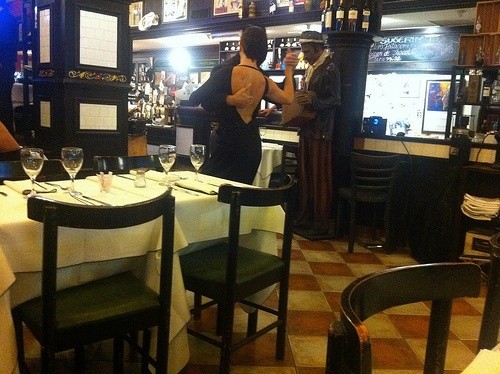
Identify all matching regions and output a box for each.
[82,196,111,206]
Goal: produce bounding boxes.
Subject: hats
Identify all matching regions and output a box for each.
[299,31,324,44]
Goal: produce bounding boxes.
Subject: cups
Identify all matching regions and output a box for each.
[99,175,112,193]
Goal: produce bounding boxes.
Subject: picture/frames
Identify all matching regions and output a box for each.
[275,0,311,10]
[421,79,461,134]
[129,0,146,30]
[161,0,191,24]
[211,0,246,19]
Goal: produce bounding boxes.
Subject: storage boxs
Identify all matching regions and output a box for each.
[468,75,483,104]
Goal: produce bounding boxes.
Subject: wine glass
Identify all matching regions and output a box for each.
[21,148,44,199]
[190,145,205,183]
[158,145,177,186]
[61,147,83,196]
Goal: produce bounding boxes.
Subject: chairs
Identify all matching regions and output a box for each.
[0,159,71,185]
[336,151,400,254]
[93,151,179,176]
[179,174,298,374]
[11,186,176,374]
[325,263,482,374]
[282,144,299,173]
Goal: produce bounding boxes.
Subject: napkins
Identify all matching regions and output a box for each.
[3,180,31,196]
[176,178,224,195]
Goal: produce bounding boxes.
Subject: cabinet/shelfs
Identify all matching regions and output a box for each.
[219,35,327,114]
[445,65,500,146]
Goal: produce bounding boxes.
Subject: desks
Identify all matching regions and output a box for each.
[0,170,285,374]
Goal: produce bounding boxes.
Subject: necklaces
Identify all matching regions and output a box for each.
[238,64,260,71]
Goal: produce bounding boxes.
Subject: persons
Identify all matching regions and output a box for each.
[293,31,342,236]
[189,21,299,186]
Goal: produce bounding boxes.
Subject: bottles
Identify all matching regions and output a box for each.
[225,38,301,51]
[495,49,500,65]
[491,86,500,104]
[458,50,465,65]
[269,0,276,15]
[304,0,312,12]
[475,46,484,65]
[449,128,471,166]
[135,169,147,188]
[289,0,294,13]
[469,133,498,163]
[475,15,481,34]
[249,2,256,17]
[322,0,371,33]
